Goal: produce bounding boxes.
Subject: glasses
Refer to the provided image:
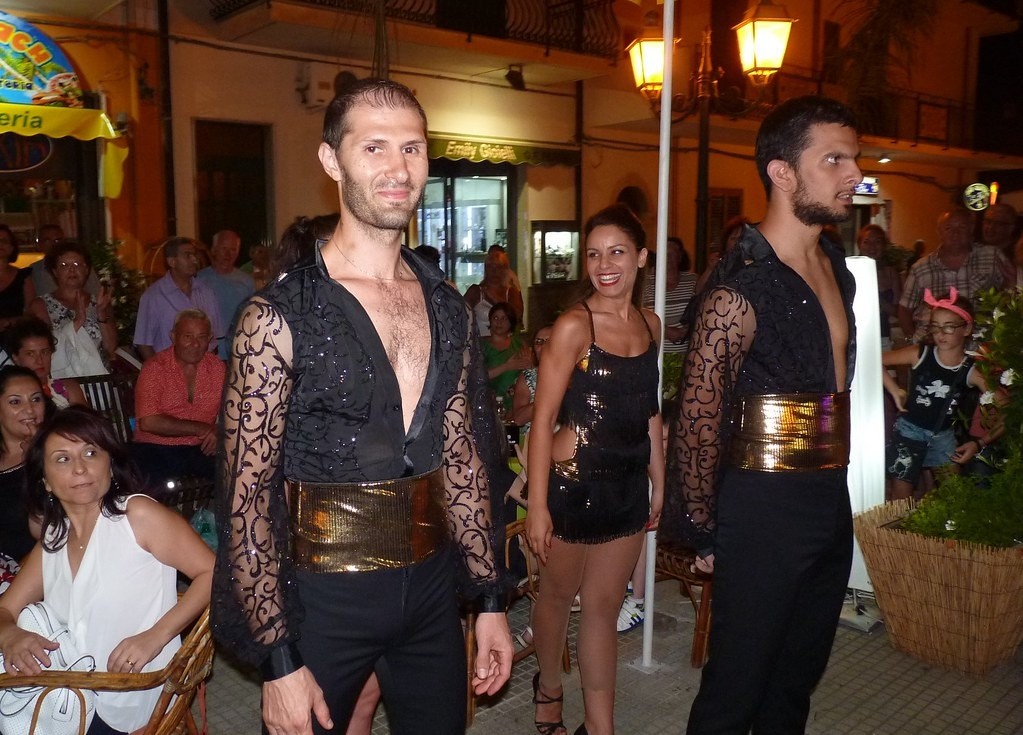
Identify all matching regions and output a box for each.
[55,260,86,270]
[531,337,548,345]
[928,320,965,334]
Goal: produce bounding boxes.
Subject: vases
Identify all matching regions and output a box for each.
[853,496,1023,682]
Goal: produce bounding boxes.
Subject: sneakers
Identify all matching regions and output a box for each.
[624,581,634,596]
[614,598,645,634]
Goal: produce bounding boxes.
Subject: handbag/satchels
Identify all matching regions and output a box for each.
[884,429,930,485]
[0,602,96,735]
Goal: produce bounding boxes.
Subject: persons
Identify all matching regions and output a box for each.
[882,287,1006,501]
[0,402,218,735]
[209,77,513,735]
[860,226,903,378]
[668,99,863,735]
[408,238,649,632]
[898,201,1023,490]
[0,220,119,571]
[135,214,383,735]
[521,205,669,735]
[618,185,752,418]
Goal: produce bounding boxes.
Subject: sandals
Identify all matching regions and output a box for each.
[516,625,533,648]
[533,672,566,735]
[571,595,582,611]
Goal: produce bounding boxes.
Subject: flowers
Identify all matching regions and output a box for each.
[896,284,1023,551]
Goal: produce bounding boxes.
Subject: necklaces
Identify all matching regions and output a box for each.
[79,543,84,550]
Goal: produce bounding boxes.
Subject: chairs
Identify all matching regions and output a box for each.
[0,372,713,735]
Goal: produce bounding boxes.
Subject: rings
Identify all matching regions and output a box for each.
[126,660,133,665]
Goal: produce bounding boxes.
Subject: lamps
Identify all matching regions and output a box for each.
[504,64,526,90]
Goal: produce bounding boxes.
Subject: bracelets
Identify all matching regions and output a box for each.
[974,439,982,453]
[97,315,111,323]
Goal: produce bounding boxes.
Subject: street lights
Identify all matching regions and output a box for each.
[621,0,798,273]
